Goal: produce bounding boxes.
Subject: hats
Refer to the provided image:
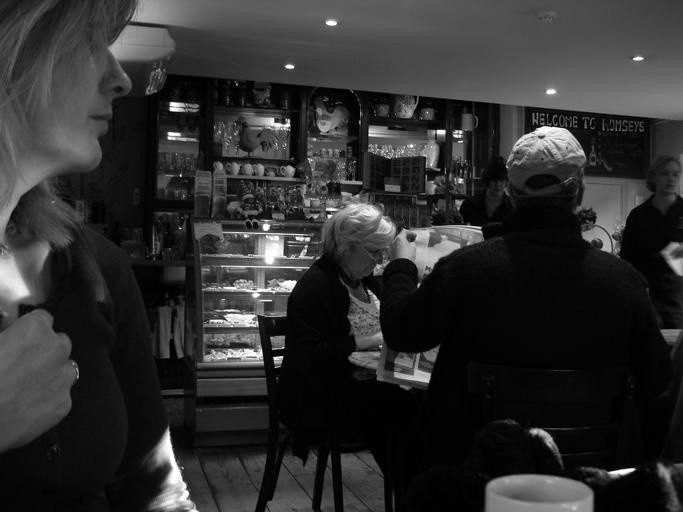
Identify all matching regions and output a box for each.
[505,124,590,197]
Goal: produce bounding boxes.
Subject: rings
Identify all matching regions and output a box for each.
[69,359,80,380]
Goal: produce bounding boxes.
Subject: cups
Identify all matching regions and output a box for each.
[160,111,490,226]
[482,473,597,511]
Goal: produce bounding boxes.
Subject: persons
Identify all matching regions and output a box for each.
[278,125,683,512]
[1,1,197,512]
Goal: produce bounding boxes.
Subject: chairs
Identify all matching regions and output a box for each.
[254,316,400,512]
